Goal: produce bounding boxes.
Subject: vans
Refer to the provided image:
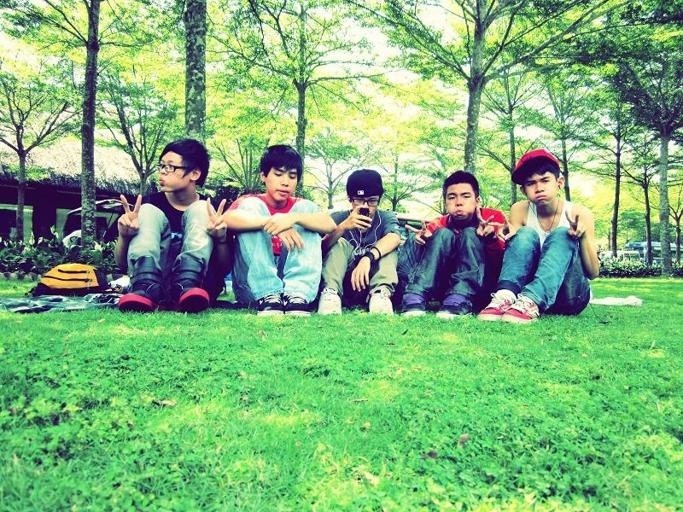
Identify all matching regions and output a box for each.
[600,251,641,262]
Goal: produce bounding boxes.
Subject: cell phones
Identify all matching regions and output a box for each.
[359,207,370,228]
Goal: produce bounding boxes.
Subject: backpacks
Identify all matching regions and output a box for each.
[32,263,111,297]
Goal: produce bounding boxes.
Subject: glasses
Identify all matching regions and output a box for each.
[157,164,184,171]
[352,197,380,206]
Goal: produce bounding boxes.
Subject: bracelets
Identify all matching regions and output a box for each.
[367,244,382,260]
[214,237,227,247]
[362,250,374,263]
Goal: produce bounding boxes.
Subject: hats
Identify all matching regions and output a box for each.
[512,150,560,185]
[346,169,384,197]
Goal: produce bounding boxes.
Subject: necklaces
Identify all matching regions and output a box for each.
[533,200,558,234]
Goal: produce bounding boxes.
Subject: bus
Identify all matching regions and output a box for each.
[625,242,683,265]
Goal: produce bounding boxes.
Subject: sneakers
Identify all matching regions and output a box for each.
[284,294,311,316]
[319,289,342,314]
[478,291,515,321]
[257,293,285,316]
[171,279,210,312]
[502,294,540,323]
[368,289,393,313]
[402,293,426,316]
[118,280,168,311]
[436,293,473,319]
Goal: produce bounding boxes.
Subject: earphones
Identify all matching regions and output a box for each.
[347,197,350,202]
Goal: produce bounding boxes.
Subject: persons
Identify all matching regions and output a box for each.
[110,138,238,318]
[397,171,509,322]
[472,149,601,322]
[219,143,340,314]
[314,167,403,316]
